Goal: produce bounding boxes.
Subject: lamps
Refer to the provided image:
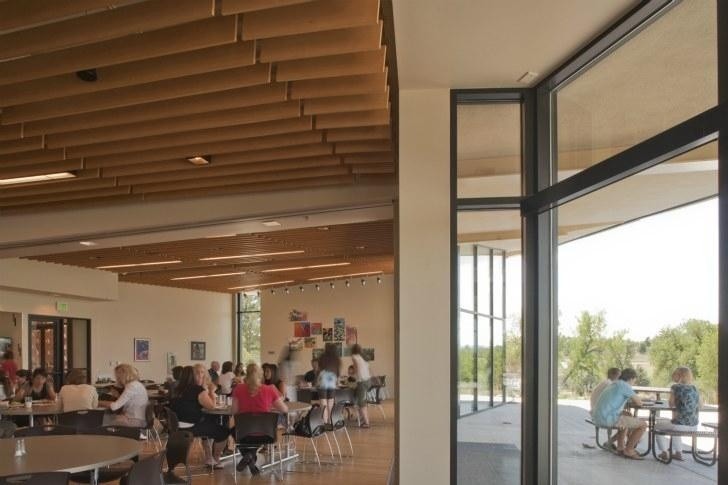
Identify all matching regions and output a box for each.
[243,274,381,298]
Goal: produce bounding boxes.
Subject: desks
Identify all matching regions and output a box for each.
[594,386,719,466]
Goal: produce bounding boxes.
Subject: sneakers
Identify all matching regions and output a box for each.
[658,451,683,461]
[236,451,261,476]
[602,441,645,461]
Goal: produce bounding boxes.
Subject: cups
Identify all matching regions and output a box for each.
[14,438,25,457]
[25,397,32,407]
[215,394,232,410]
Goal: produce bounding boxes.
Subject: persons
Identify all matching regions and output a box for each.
[164,344,371,476]
[1,350,152,443]
[589,368,637,452]
[654,367,700,460]
[592,368,647,460]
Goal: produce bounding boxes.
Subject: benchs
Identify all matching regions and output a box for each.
[584,417,718,437]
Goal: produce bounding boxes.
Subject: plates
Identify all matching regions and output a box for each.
[10,400,51,408]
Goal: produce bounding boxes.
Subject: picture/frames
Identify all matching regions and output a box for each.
[135,338,151,361]
[191,341,206,360]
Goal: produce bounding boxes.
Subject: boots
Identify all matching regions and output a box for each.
[347,413,371,427]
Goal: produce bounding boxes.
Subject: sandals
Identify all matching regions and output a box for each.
[204,458,225,469]
